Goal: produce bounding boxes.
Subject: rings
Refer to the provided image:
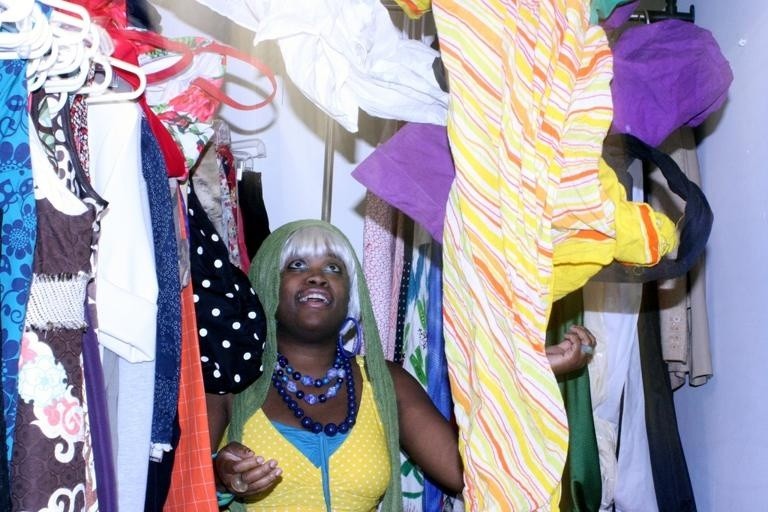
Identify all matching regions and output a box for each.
[231,475,250,494]
[580,345,593,357]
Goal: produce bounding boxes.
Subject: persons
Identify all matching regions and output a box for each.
[205,219,596,512]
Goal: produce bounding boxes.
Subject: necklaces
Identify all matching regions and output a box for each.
[271,342,356,435]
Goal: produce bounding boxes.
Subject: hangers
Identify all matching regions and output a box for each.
[0,0,145,106]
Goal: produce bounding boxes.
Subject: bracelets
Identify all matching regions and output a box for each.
[211,452,234,506]
